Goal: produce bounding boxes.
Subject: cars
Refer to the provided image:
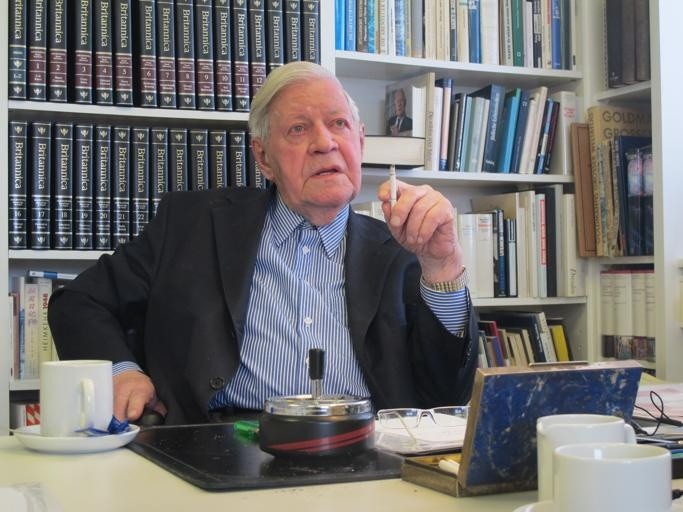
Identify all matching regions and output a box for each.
[537,413,672,512]
[40,360,113,436]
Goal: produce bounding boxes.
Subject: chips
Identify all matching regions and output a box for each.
[234,421,260,439]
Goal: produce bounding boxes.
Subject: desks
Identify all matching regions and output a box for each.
[0,371,682,511]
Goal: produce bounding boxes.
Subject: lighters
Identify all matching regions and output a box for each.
[234,421,260,439]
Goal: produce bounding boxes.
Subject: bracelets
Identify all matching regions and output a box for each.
[256,397,374,464]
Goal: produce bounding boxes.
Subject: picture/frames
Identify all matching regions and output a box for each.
[0,371,682,511]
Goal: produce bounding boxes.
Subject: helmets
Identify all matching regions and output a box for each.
[15,425,139,454]
[373,413,473,456]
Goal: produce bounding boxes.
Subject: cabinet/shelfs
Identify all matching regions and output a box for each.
[5,259,40,435]
[585,1,665,383]
[4,0,329,259]
[453,178,596,363]
[328,2,592,183]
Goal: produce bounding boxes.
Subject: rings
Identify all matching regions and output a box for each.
[432,457,459,475]
[233,421,259,435]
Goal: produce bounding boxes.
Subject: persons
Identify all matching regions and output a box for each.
[47,60,478,432]
[386,89,412,138]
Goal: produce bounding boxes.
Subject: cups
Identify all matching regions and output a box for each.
[376,405,468,446]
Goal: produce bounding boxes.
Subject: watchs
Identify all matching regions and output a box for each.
[420,267,471,293]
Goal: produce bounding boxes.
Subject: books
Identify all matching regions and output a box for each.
[335,1,577,70]
[599,270,655,362]
[362,134,426,169]
[9,1,320,113]
[454,184,589,299]
[606,1,651,88]
[9,122,276,250]
[477,311,575,368]
[459,359,643,489]
[350,201,387,222]
[571,106,656,258]
[10,400,41,429]
[9,269,79,380]
[382,70,578,176]
